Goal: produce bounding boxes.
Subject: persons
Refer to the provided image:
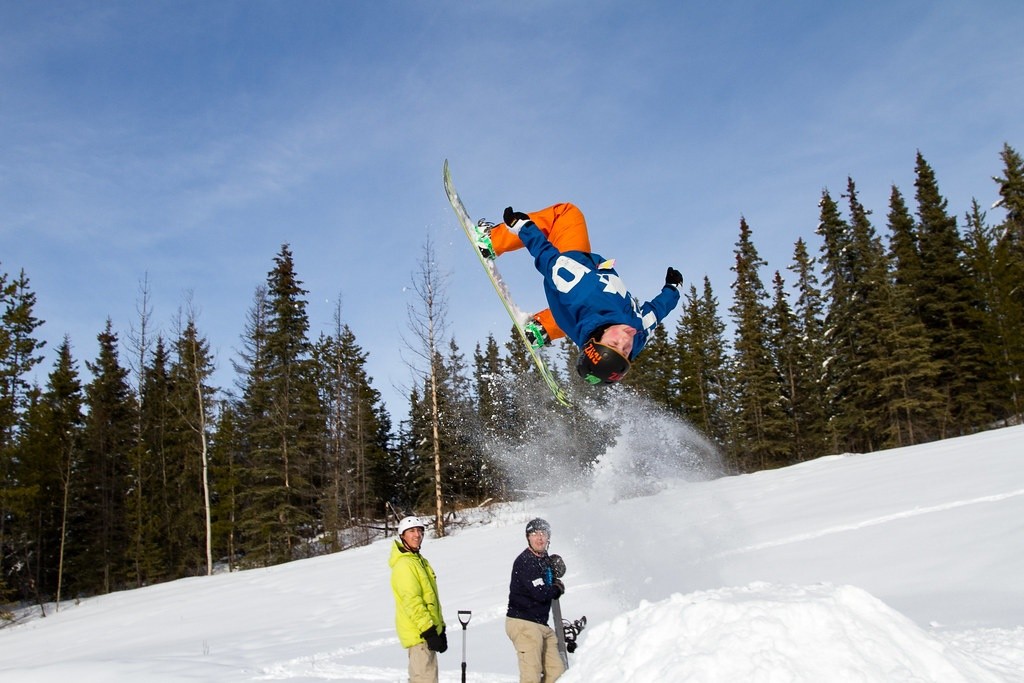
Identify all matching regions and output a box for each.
[389,516,448,683]
[505,518,566,683]
[477,202,686,387]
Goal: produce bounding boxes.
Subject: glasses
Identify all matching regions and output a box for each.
[528,531,547,537]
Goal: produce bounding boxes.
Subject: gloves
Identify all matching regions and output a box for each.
[667,266,684,294]
[424,630,441,652]
[503,206,530,236]
[438,631,448,653]
[551,554,566,578]
[553,579,565,594]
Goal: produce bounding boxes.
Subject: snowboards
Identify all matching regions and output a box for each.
[441,158,575,409]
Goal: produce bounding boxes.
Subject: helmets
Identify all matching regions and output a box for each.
[577,338,631,387]
[526,518,552,537]
[398,516,425,536]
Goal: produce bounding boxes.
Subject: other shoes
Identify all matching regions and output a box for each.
[477,221,496,258]
[526,320,548,346]
